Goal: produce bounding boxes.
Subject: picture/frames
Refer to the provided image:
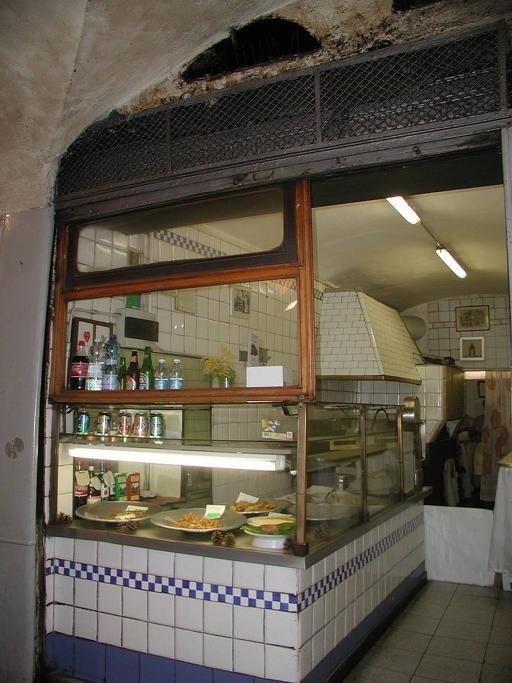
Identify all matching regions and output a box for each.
[458,334,486,362]
[454,303,492,332]
[228,282,252,319]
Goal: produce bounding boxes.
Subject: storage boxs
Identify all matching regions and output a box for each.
[245,364,293,403]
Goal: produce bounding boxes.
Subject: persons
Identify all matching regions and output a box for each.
[471,413,491,507]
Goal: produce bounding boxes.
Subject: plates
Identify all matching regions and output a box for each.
[148,507,244,532]
[76,501,162,522]
[230,496,282,513]
[288,500,358,521]
[243,519,289,538]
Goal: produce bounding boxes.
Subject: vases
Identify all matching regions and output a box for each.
[218,377,234,390]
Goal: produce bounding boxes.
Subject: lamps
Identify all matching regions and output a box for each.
[383,194,423,226]
[431,242,471,280]
[67,444,290,473]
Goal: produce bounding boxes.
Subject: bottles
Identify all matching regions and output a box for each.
[70,333,185,390]
[74,458,115,506]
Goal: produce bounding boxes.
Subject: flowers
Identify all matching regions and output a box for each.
[201,347,237,379]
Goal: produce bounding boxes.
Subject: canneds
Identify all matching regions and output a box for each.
[76,434,163,445]
[74,411,91,435]
[119,412,164,436]
[96,411,111,434]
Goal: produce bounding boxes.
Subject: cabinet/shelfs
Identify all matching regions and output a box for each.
[290,395,424,555]
[62,403,299,552]
